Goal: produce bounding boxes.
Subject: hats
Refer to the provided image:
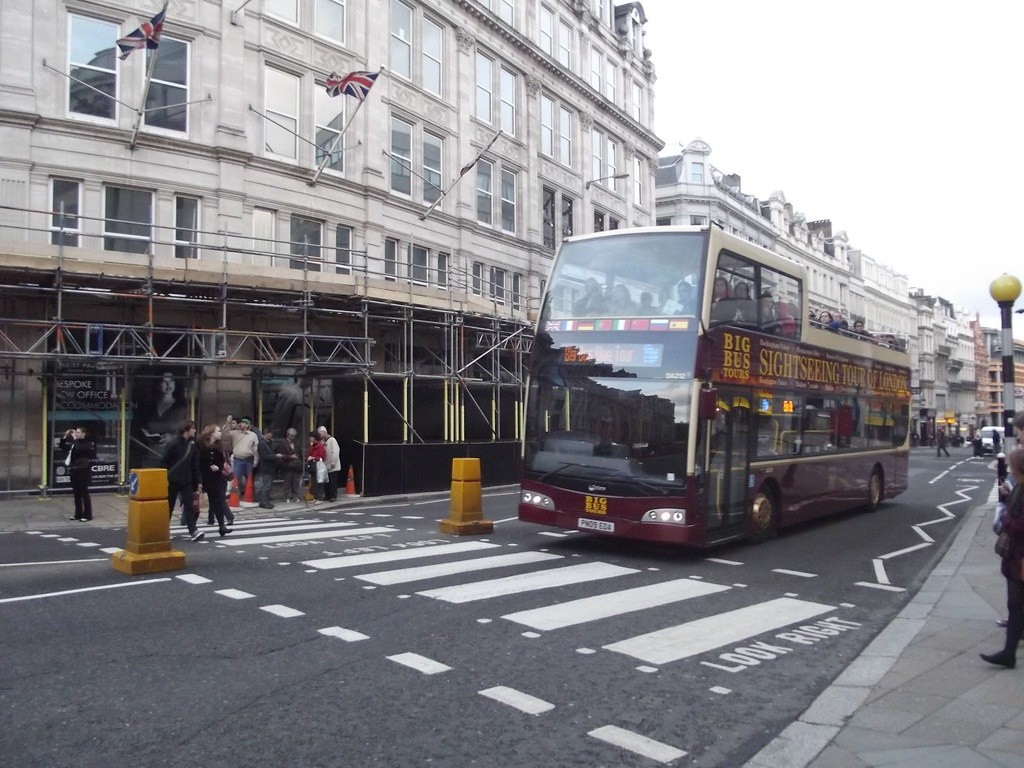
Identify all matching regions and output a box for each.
[242,419,250,425]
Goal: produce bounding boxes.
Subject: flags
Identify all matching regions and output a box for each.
[117,11,165,60]
[326,70,379,101]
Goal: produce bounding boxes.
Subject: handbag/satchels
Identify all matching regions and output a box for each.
[192,491,200,512]
[221,463,233,475]
[995,531,1010,558]
[316,456,329,483]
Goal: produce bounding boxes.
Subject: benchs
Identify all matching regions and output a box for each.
[779,430,831,457]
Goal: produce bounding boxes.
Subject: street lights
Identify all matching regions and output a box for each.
[990,273,1021,469]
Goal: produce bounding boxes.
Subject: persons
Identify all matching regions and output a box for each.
[60,427,92,522]
[574,276,871,340]
[937,430,949,457]
[162,415,341,541]
[139,372,182,467]
[979,410,1024,669]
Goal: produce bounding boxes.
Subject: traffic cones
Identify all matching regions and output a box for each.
[240,471,259,508]
[343,465,360,498]
[227,475,245,512]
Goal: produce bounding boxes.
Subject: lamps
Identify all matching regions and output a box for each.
[586,172,629,189]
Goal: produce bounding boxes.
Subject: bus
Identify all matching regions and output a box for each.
[519,220,913,547]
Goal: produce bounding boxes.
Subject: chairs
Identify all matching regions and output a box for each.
[768,419,778,456]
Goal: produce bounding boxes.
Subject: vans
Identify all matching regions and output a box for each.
[981,426,1005,454]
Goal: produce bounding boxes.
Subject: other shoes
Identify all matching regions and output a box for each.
[286,499,290,504]
[997,618,1008,626]
[292,497,300,503]
[191,532,205,541]
[315,500,323,505]
[330,498,337,502]
[979,649,1016,668]
[80,518,88,522]
[227,519,233,525]
[70,516,76,520]
[218,525,233,536]
[261,504,275,509]
